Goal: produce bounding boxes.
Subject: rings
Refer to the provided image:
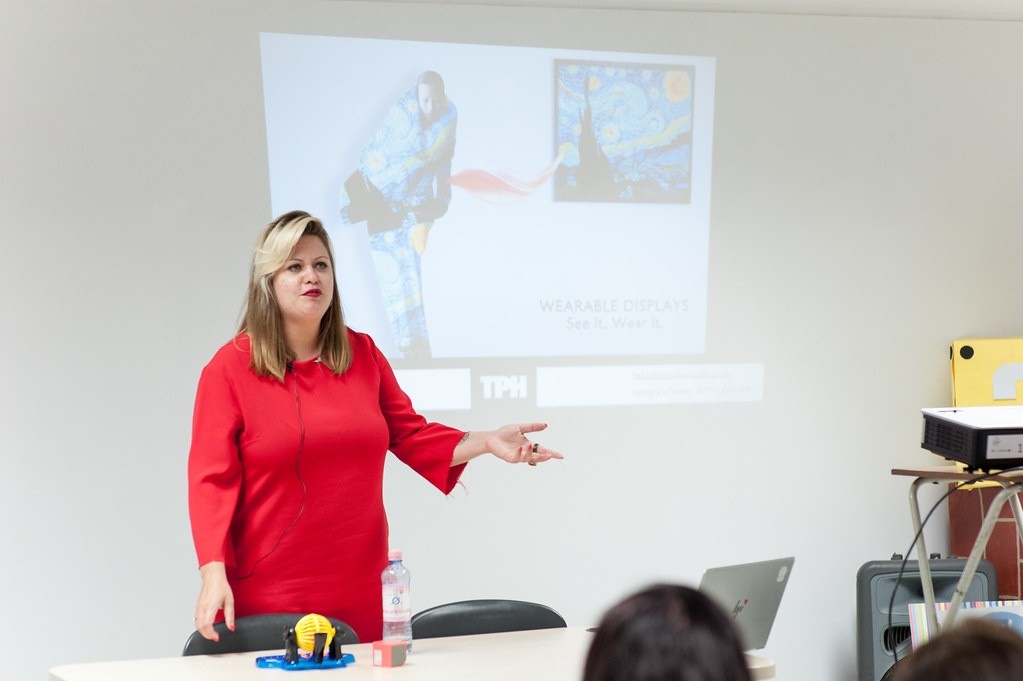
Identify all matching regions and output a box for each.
[193,618,197,621]
[533,443,539,453]
[528,461,536,466]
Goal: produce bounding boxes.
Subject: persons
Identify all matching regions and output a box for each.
[188,211,564,644]
[583,585,755,681]
[890,616,1023,681]
[361,70,458,258]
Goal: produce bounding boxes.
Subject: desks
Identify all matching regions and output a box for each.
[890,467,1022,643]
[48,626,776,681]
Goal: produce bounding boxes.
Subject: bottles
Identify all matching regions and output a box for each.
[381,551,413,654]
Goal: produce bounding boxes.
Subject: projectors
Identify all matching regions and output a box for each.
[921,406,1023,473]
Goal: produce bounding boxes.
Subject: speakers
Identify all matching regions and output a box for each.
[857,553,999,681]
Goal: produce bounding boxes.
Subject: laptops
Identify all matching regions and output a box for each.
[696,557,796,651]
